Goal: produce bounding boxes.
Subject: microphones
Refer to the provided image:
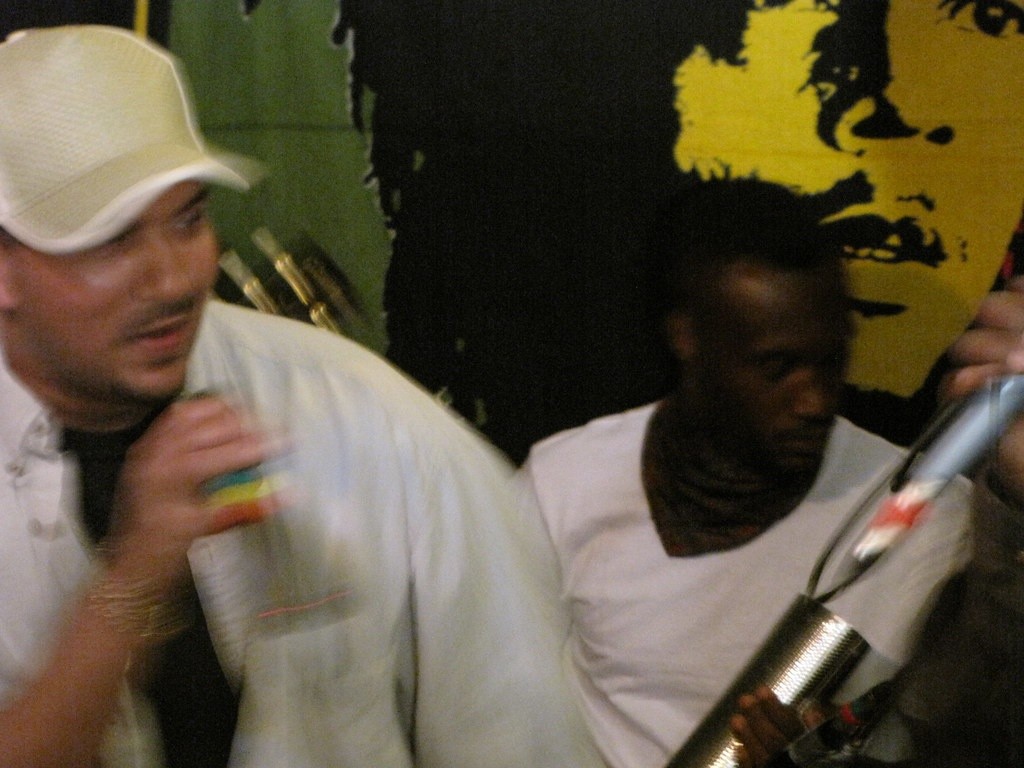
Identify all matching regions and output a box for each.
[812,372,1024,605]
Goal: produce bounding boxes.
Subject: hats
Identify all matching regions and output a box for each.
[0,27,251,257]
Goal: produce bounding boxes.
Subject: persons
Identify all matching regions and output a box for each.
[0,28,828,767]
[842,278,1024,768]
[501,177,979,768]
